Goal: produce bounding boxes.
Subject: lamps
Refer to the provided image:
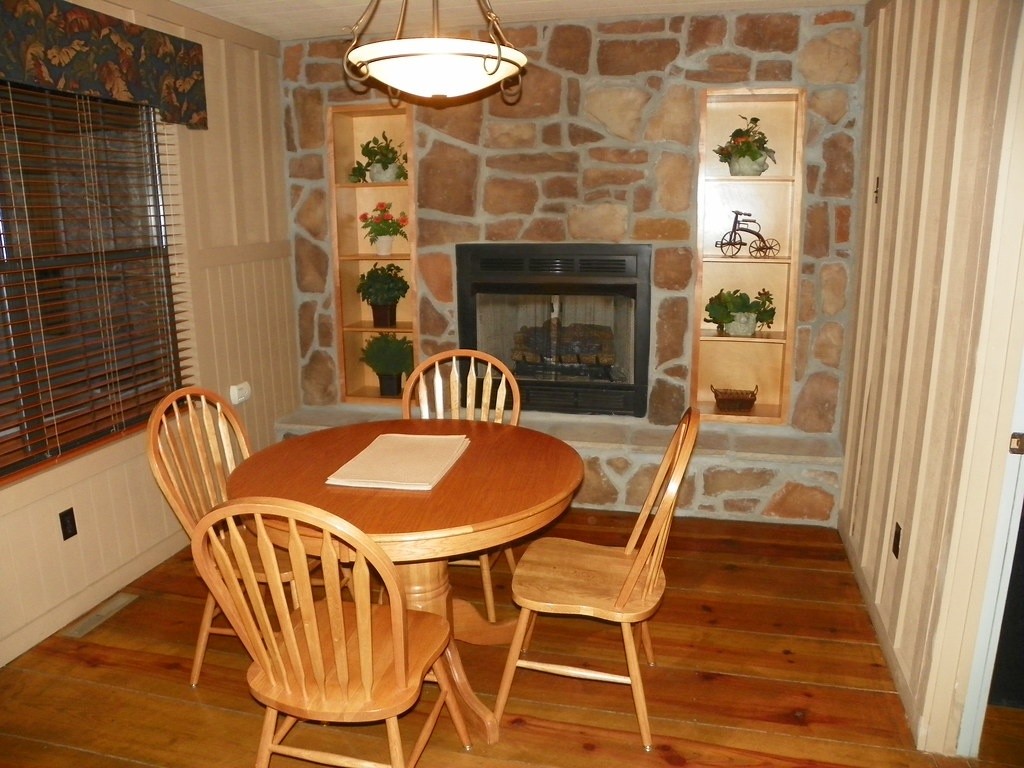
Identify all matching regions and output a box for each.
[340,0,528,99]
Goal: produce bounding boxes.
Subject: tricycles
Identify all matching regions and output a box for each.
[715,210,781,258]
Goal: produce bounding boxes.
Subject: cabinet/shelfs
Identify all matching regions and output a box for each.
[326,101,419,406]
[691,85,804,428]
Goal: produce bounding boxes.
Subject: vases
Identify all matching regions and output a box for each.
[728,151,769,176]
[725,313,757,337]
[374,235,393,257]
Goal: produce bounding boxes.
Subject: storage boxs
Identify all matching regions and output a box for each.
[709,383,758,410]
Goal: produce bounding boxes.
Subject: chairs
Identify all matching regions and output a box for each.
[146,385,356,689]
[378,348,521,623]
[189,496,473,768]
[495,406,702,751]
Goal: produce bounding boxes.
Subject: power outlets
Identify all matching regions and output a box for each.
[58,507,78,542]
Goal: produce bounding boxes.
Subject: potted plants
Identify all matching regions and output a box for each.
[348,130,408,183]
[357,262,409,326]
[357,332,413,396]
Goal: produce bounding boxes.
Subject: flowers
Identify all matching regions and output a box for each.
[359,202,409,245]
[704,288,777,333]
[713,114,776,163]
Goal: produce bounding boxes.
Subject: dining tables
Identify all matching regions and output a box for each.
[225,419,584,745]
